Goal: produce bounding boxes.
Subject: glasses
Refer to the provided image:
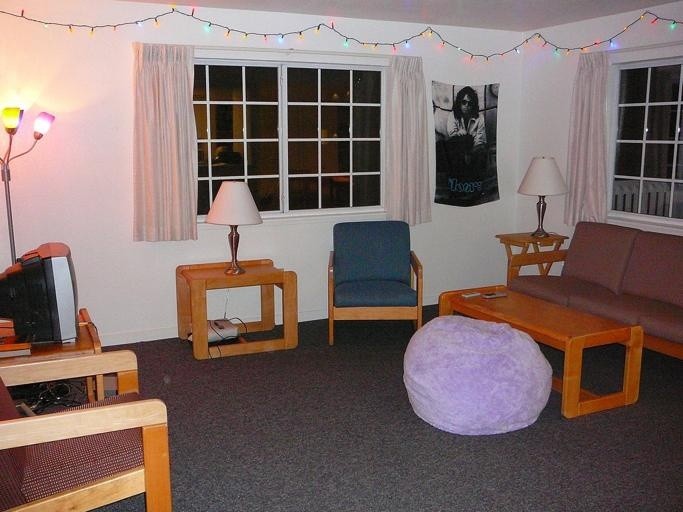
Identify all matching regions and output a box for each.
[462,99,475,105]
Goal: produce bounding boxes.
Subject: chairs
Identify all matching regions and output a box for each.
[2,347,172,512]
[328,221,423,346]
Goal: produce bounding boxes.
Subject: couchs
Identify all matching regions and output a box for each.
[509,222,683,359]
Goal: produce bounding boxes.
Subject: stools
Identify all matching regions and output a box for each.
[403,314,554,436]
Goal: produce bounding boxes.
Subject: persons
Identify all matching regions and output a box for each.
[446,86,487,147]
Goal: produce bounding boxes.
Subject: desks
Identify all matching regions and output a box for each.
[176,259,298,360]
[495,232,569,286]
[438,285,644,419]
[1,308,104,402]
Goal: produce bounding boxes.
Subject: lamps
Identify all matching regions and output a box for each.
[517,157,570,237]
[205,181,263,274]
[0,108,55,264]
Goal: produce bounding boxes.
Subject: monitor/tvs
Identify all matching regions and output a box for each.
[0,242,79,344]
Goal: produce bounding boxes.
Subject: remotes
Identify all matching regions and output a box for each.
[482,292,506,298]
[461,292,480,297]
